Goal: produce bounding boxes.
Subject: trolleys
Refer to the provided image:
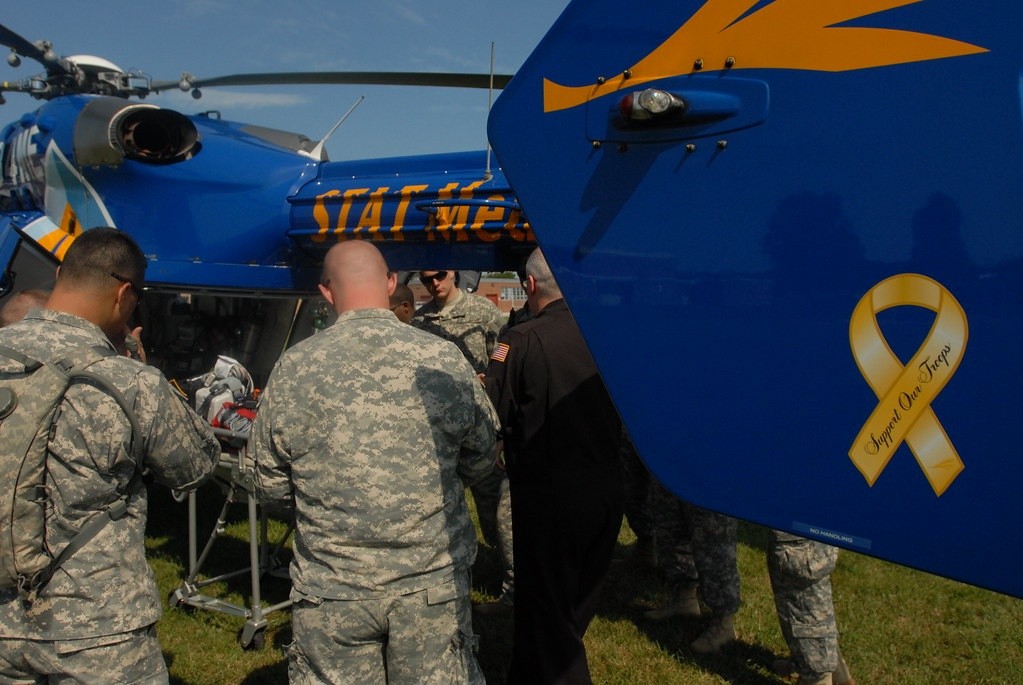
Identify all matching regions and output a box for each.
[163,421,297,651]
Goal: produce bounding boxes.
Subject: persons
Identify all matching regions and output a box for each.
[243,239,504,685]
[388,246,838,685]
[0,288,52,328]
[0,226,219,685]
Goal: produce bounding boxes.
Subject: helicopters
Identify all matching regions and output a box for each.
[1,0,1022,599]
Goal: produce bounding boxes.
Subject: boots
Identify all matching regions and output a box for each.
[774,658,802,678]
[795,671,834,685]
[690,610,736,653]
[645,583,701,619]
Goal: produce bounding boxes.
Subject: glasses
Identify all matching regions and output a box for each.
[111,272,146,306]
[420,270,448,284]
